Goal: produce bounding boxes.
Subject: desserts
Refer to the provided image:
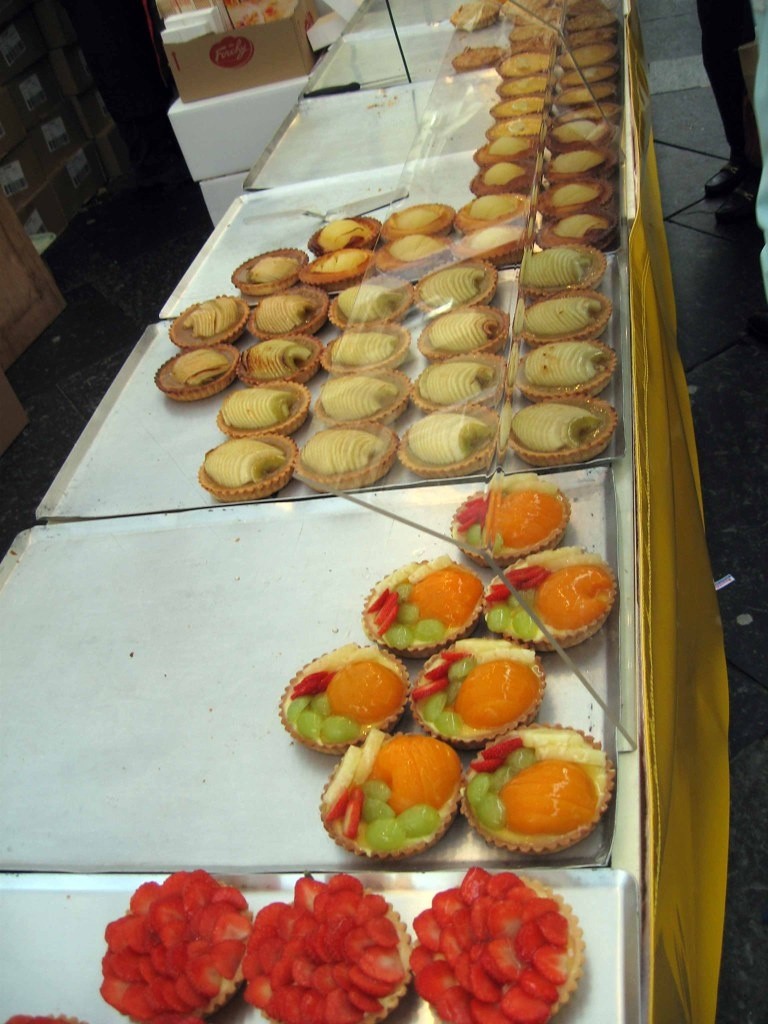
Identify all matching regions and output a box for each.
[157,122,618,503]
[277,468,616,860]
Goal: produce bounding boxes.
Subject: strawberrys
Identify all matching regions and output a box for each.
[100,865,574,1024]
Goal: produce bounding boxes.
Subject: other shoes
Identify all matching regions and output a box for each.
[705,160,745,200]
[715,182,759,224]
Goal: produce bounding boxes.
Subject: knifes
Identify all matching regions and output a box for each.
[302,75,409,99]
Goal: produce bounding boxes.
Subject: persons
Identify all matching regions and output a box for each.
[695,0,762,221]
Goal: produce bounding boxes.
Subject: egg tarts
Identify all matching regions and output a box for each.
[452,0,621,139]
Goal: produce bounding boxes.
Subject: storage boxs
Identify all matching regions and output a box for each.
[168,75,309,182]
[0,0,127,256]
[199,170,246,229]
[163,1,321,103]
